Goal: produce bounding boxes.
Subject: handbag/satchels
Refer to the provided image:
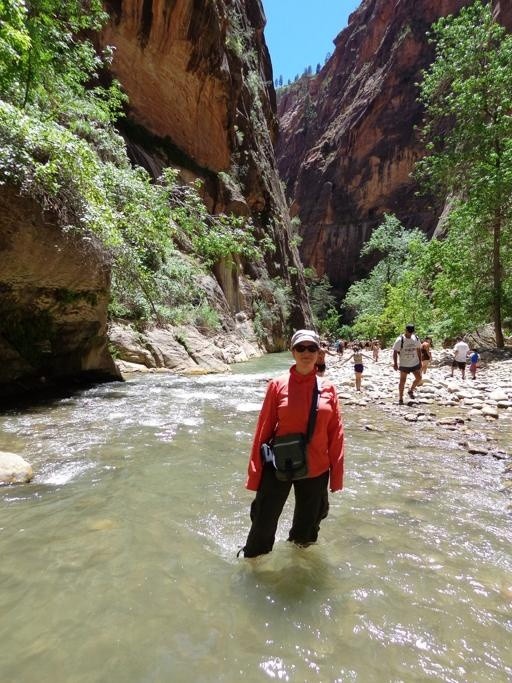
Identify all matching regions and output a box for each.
[271,376,322,483]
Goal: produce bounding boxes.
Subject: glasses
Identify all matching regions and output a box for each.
[295,344,318,353]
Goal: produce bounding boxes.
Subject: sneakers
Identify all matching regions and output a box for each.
[451,374,476,380]
[407,390,414,399]
[398,400,404,405]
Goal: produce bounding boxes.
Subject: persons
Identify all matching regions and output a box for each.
[421,337,433,375]
[240,330,348,558]
[469,349,481,380]
[450,336,470,380]
[315,338,381,392]
[392,323,423,405]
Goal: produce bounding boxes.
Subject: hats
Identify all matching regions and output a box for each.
[406,324,414,331]
